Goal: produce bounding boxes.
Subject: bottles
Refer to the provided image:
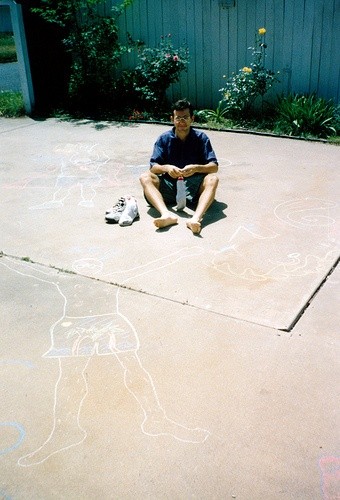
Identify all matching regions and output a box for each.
[177,176,186,208]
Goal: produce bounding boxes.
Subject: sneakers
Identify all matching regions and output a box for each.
[105,197,139,222]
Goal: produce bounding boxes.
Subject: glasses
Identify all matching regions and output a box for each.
[174,116,190,120]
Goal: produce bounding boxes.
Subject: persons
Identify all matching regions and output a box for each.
[139,99,220,234]
